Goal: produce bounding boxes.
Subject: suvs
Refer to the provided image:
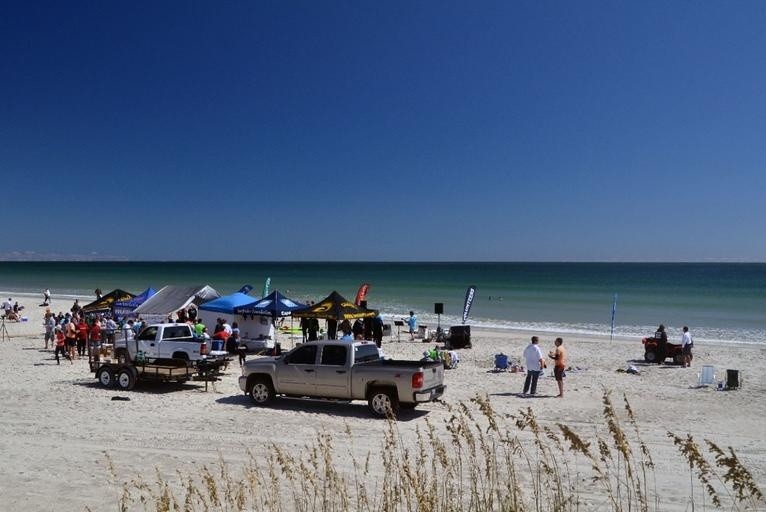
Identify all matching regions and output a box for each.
[641,336,694,366]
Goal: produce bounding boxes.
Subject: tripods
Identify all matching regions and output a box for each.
[0,318,10,342]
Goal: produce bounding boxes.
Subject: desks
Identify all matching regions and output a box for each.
[209,351,229,358]
[189,358,233,391]
[240,339,274,355]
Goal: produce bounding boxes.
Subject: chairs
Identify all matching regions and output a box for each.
[697,365,717,387]
[725,369,742,389]
[495,355,508,371]
[383,324,392,342]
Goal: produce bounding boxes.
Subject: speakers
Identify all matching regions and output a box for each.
[450,326,470,349]
[435,303,443,314]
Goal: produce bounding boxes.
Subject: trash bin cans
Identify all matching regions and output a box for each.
[418,326,428,338]
[211,340,222,350]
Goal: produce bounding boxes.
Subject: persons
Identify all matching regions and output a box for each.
[167,304,240,356]
[523,336,544,394]
[401,312,417,341]
[1,298,25,323]
[548,337,566,397]
[654,324,667,364]
[300,311,384,347]
[539,358,547,376]
[421,345,459,369]
[43,287,148,365]
[681,326,693,368]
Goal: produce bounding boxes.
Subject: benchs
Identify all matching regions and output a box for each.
[146,357,188,375]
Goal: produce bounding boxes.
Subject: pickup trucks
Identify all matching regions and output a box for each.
[112,323,230,365]
[237,338,446,417]
